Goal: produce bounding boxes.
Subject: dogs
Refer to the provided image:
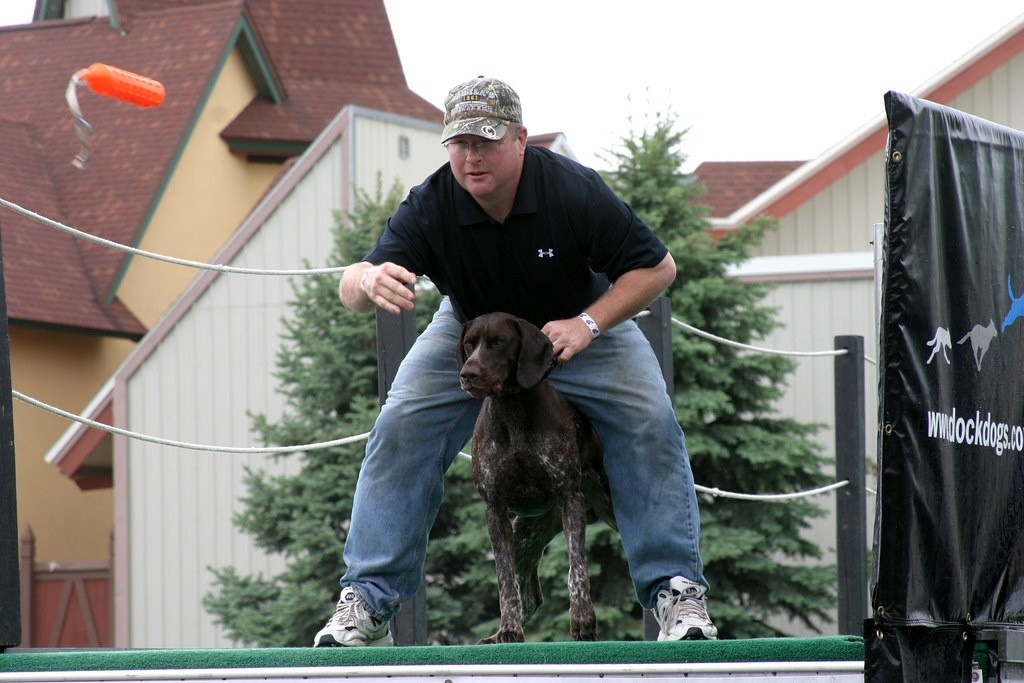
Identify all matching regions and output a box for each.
[454,312,618,646]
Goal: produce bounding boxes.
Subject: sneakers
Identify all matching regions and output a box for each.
[314,587,393,647]
[650,576,718,641]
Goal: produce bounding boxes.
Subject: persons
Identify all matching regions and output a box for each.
[312,75,717,648]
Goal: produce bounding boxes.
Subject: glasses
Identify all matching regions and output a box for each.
[443,128,521,155]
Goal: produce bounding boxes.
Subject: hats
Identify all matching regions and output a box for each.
[440,75,522,144]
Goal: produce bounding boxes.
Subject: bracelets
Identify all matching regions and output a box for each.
[578,312,600,338]
[361,271,374,290]
